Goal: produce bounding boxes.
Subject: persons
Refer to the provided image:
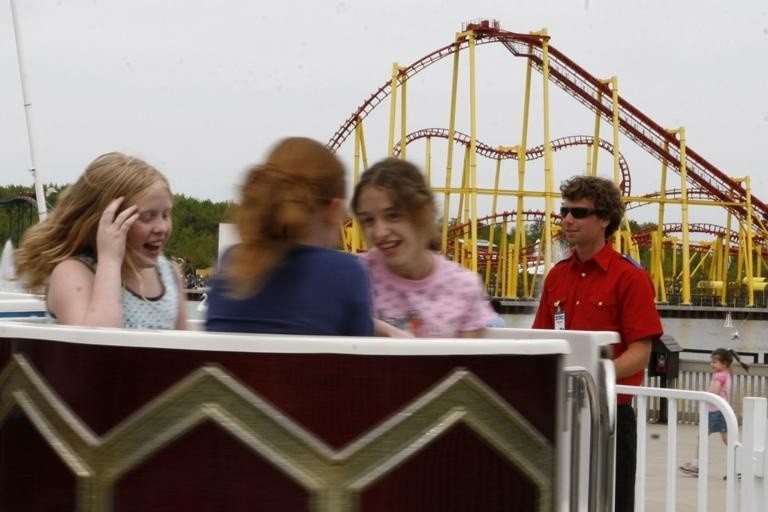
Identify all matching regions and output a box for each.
[171,252,205,290]
[204,135,377,336]
[482,297,507,328]
[531,174,664,510]
[11,150,192,332]
[348,156,497,339]
[677,346,752,482]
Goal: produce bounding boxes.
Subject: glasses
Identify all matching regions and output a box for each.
[559,207,604,219]
